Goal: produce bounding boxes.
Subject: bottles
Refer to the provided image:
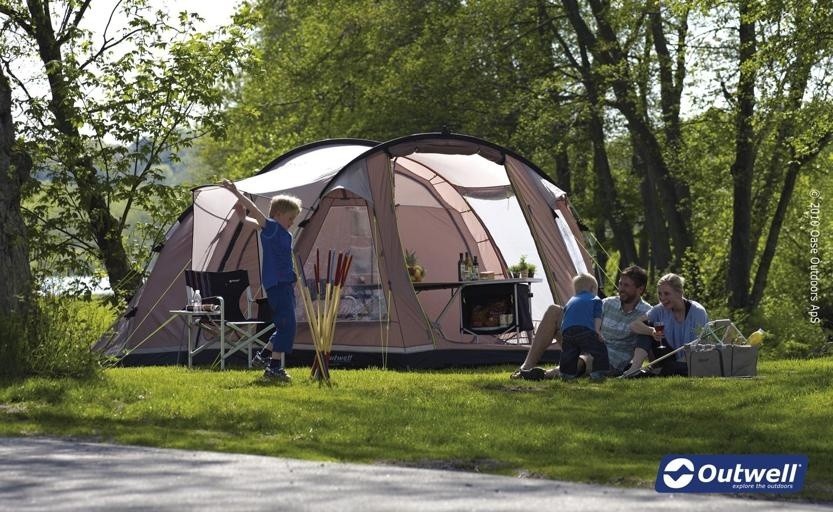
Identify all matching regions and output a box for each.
[458,251,479,281]
[193,290,202,312]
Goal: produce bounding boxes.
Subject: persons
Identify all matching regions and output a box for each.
[218,179,301,383]
[509,265,653,379]
[562,272,608,382]
[626,272,707,378]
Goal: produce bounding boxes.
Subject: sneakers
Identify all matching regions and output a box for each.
[561,376,578,384]
[590,372,604,381]
[252,352,270,367]
[262,366,291,381]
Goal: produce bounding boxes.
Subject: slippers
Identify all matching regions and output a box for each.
[510,370,522,379]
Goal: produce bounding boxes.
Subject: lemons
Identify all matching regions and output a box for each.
[410,265,424,282]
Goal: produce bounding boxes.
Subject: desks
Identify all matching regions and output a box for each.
[345,282,475,346]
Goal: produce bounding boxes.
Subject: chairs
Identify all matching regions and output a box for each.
[168,269,285,371]
[305,278,357,319]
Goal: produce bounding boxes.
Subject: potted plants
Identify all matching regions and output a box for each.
[508,265,520,277]
[518,256,528,277]
[528,264,535,277]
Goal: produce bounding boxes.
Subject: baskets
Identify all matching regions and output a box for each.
[684,318,759,377]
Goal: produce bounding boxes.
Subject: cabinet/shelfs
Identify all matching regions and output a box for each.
[457,278,542,346]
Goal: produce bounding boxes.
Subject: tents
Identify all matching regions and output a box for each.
[213,133,596,370]
[86,136,382,365]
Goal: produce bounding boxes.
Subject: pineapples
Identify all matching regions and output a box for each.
[405,247,418,275]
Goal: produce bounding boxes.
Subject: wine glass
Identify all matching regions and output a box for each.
[653,321,667,349]
